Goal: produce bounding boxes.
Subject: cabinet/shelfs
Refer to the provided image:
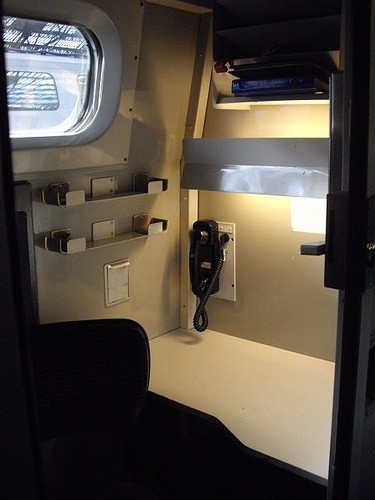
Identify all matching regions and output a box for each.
[35,169,171,256]
